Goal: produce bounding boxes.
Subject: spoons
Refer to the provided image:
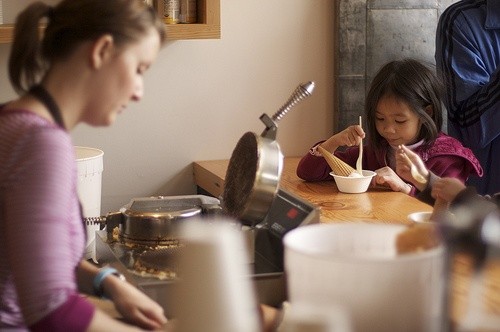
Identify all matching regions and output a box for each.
[398,144,428,183]
[356,116,363,173]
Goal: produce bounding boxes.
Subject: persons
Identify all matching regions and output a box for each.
[434,0,500,196]
[0,0,172,332]
[294,54,483,205]
[389,176,500,258]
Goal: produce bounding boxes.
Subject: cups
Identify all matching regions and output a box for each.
[282,223,447,332]
[174,217,260,332]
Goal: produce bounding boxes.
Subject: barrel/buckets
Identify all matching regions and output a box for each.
[74,146,104,260]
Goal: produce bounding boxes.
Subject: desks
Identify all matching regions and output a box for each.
[193,157,500,332]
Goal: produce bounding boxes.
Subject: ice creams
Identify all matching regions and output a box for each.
[317,145,362,177]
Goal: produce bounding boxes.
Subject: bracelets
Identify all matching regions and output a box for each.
[91,265,125,294]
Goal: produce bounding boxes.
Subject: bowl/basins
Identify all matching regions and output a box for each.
[409,212,454,228]
[329,170,377,193]
